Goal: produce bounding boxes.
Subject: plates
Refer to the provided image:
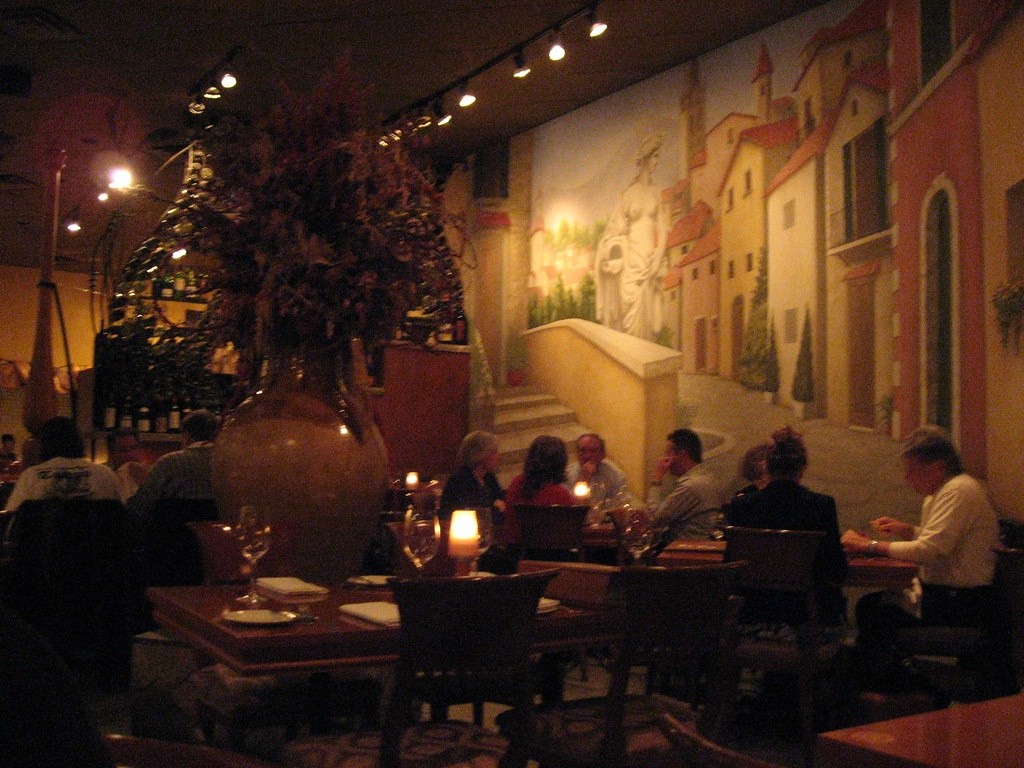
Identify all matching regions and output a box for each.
[346,574,398,585]
[225,607,299,622]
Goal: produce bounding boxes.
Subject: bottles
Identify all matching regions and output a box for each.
[453,308,468,345]
[102,386,193,433]
[143,264,211,301]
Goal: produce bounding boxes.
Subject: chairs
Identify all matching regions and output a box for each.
[0,482,1024,768]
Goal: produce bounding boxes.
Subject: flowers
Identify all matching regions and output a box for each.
[99,45,477,434]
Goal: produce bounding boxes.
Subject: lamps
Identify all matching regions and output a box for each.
[459,79,476,107]
[381,96,453,146]
[512,50,532,78]
[189,60,237,114]
[548,26,565,61]
[575,482,588,496]
[405,472,418,490]
[447,510,480,556]
[585,6,608,38]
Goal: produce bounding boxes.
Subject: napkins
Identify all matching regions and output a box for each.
[257,577,330,595]
[360,576,395,584]
[340,601,401,626]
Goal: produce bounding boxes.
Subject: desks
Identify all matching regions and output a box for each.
[493,525,621,547]
[662,550,919,725]
[150,583,617,752]
[818,693,1024,768]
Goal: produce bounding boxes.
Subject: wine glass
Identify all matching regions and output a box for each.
[235,504,271,610]
[621,508,649,566]
[403,508,441,576]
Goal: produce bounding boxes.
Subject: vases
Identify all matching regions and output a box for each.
[210,322,390,587]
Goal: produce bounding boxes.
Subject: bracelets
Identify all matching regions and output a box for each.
[647,481,662,487]
[870,541,878,554]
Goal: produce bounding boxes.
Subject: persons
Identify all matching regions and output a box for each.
[841,423,997,712]
[0,408,218,513]
[440,425,847,736]
